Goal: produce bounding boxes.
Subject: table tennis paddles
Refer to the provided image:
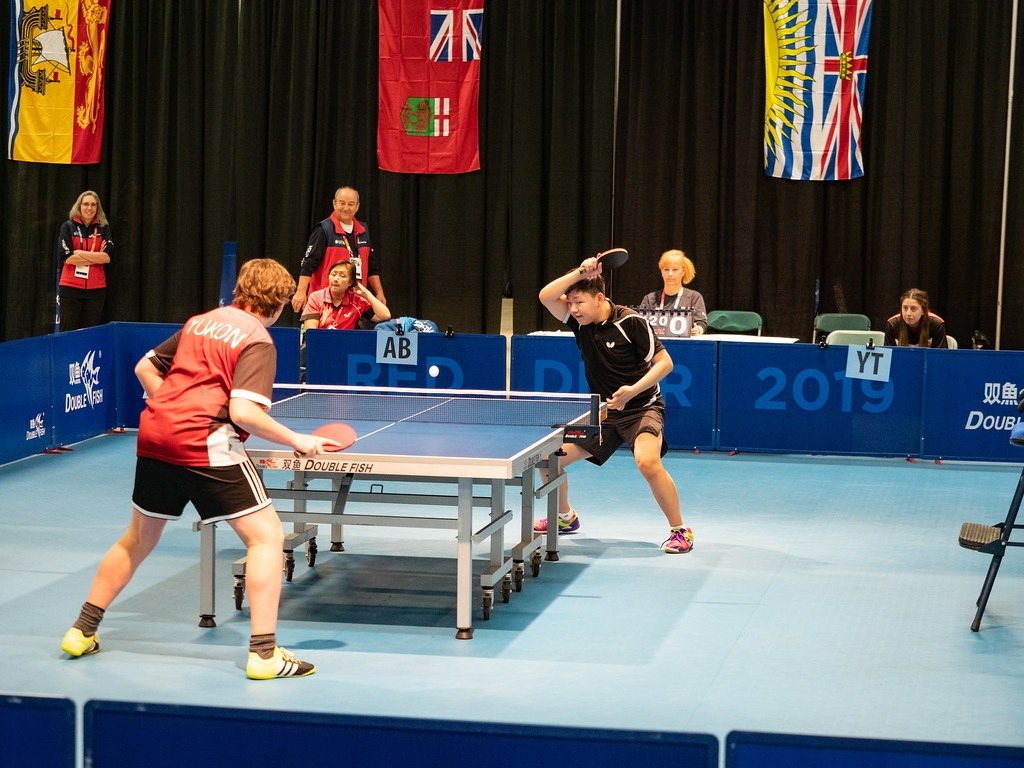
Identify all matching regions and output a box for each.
[293,423,357,459]
[579,248,630,274]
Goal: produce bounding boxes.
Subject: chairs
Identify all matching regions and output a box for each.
[706,310,761,336]
[813,312,958,350]
[1010,390,1024,446]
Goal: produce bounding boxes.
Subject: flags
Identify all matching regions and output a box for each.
[763,0,872,180]
[8,0,112,164]
[379,0,484,174]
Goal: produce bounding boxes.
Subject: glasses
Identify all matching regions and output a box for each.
[80,202,98,207]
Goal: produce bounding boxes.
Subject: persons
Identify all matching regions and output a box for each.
[639,250,708,336]
[885,288,949,348]
[534,254,693,554]
[299,260,391,383]
[61,259,341,679]
[59,191,114,331]
[291,187,386,313]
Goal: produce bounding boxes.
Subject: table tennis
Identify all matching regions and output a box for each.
[428,365,440,377]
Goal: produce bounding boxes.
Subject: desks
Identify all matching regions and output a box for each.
[526,330,801,344]
[196,384,609,640]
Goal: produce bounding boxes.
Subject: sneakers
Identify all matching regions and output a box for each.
[663,527,695,553]
[246,645,316,680]
[531,509,580,534]
[60,626,103,657]
